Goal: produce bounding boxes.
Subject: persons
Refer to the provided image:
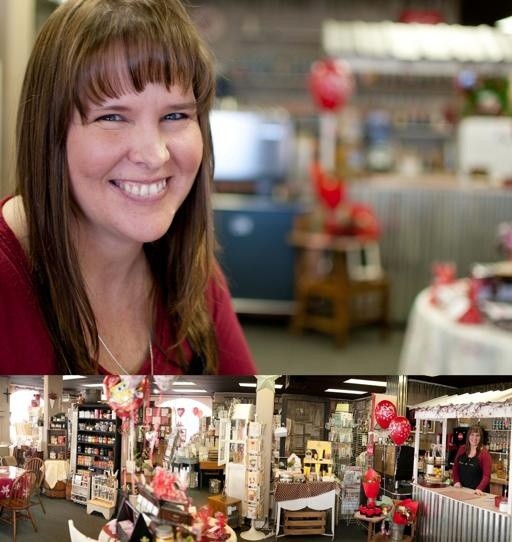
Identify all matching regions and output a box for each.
[0,0,258,377]
[451,424,494,497]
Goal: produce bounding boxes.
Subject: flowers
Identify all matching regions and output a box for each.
[376,496,418,525]
[358,468,382,517]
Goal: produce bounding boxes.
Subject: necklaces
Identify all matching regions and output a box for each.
[82,257,157,376]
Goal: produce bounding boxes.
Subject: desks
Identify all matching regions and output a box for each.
[275,482,336,536]
[348,173,512,325]
[172,456,199,492]
[209,193,295,315]
[200,461,224,490]
[410,483,512,542]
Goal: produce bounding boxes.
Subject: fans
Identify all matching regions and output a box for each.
[399,279,512,376]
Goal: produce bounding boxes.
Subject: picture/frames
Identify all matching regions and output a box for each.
[406,405,435,433]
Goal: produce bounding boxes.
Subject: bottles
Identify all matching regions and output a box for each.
[77,434,115,456]
[488,435,507,453]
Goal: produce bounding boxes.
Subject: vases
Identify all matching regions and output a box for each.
[98,516,237,542]
[389,522,405,540]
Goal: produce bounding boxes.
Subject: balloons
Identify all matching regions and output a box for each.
[388,415,413,447]
[375,399,399,430]
[306,55,360,114]
[100,376,204,443]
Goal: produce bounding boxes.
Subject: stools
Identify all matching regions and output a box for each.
[354,511,385,542]
[86,500,115,520]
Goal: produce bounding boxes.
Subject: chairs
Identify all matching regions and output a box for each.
[68,520,98,542]
[292,213,391,350]
[0,456,46,542]
[370,499,415,542]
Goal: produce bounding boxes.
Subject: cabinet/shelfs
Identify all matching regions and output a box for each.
[218,404,255,515]
[47,405,122,505]
[484,429,511,455]
[208,496,241,532]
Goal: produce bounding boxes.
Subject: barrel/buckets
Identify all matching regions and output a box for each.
[45,459,67,499]
